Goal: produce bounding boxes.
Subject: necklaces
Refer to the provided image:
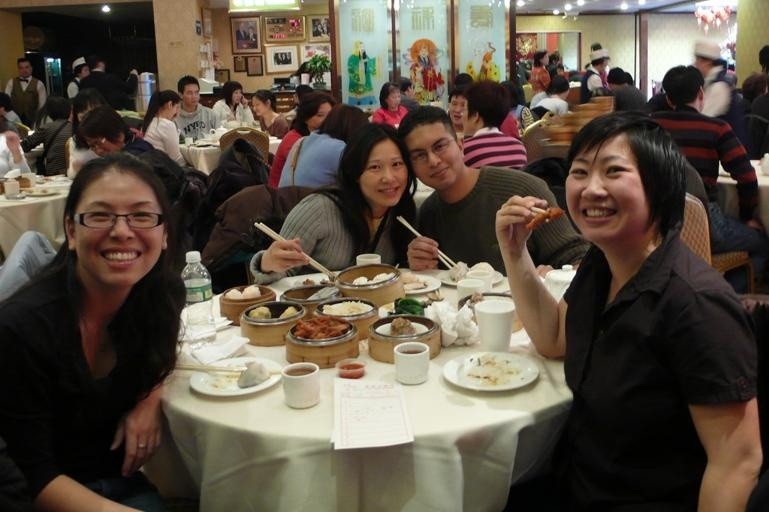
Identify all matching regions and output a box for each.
[80,316,96,372]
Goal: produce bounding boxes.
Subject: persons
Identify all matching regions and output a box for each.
[0,39,768,296]
[235,22,247,43]
[0,149,190,512]
[246,27,257,42]
[312,24,324,37]
[321,19,330,34]
[492,107,768,512]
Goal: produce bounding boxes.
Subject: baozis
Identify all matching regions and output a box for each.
[280,306,297,318]
[249,306,271,319]
[225,286,241,300]
[242,285,261,299]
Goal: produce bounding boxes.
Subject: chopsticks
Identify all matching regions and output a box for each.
[174,362,281,375]
[394,213,461,275]
[531,205,553,224]
[252,217,337,283]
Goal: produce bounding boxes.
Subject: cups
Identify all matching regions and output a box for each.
[545,268,580,298]
[394,342,431,386]
[458,268,493,303]
[473,298,516,353]
[334,358,368,379]
[356,254,382,267]
[281,361,323,410]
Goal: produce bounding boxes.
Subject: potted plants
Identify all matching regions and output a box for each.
[305,52,333,90]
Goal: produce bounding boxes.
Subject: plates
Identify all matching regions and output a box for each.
[322,302,374,315]
[399,275,441,295]
[377,296,456,323]
[189,357,285,398]
[0,170,74,200]
[437,266,506,288]
[441,352,540,393]
[181,297,236,331]
[182,120,280,147]
[376,322,429,337]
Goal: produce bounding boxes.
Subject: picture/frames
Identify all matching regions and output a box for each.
[201,6,213,39]
[215,68,230,84]
[228,14,332,77]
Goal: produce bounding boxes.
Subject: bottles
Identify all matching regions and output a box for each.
[180,249,217,347]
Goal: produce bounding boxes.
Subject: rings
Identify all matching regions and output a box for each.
[138,443,146,449]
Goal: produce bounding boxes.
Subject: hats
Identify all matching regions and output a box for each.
[589,49,608,61]
[695,43,721,61]
[72,57,85,69]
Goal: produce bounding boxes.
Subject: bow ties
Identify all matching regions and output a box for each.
[17,77,27,83]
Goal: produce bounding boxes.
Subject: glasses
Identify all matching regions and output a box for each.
[74,211,164,229]
[89,137,106,151]
[410,140,454,161]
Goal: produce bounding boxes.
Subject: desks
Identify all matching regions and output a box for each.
[0,169,83,263]
[176,134,287,178]
[684,149,769,237]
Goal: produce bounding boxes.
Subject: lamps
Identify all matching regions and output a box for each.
[692,0,735,35]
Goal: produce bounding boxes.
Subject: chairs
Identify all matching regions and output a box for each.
[217,128,274,169]
[683,192,754,306]
[514,114,550,166]
[729,292,768,511]
